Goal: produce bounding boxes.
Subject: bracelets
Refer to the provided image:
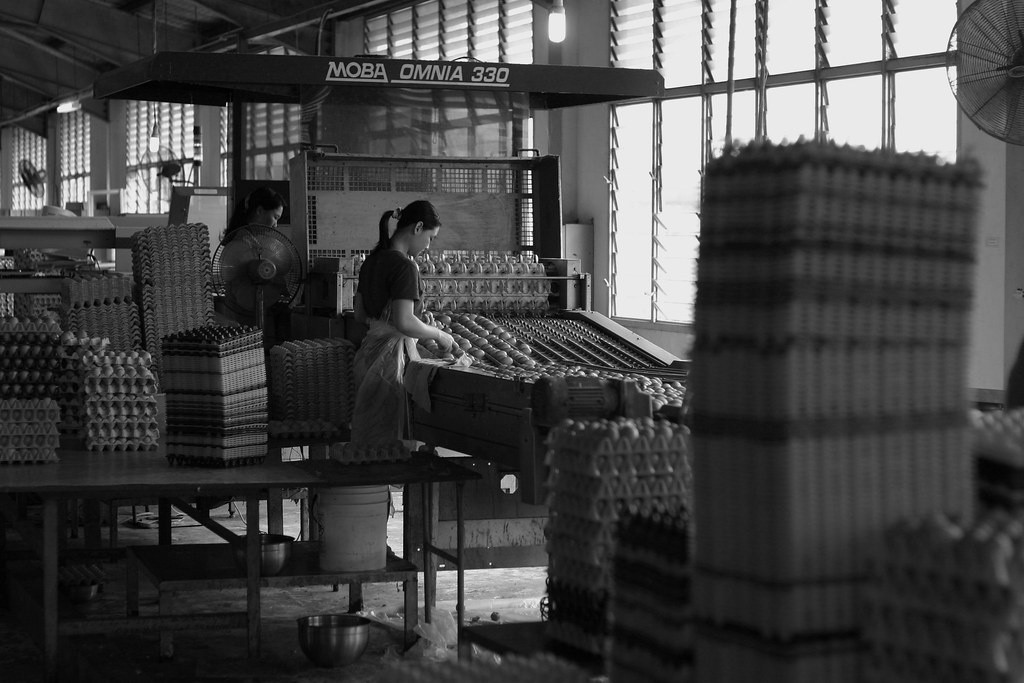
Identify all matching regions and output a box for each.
[435,329,441,343]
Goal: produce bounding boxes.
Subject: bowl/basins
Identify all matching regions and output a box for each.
[231,534,295,577]
[38,584,100,603]
[296,614,372,667]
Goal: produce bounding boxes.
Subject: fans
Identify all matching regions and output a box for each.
[946,0,1023,148]
[140,146,185,202]
[19,160,47,198]
[212,224,307,335]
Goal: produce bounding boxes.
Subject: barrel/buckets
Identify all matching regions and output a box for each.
[311,485,389,572]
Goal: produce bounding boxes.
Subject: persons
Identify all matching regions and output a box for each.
[350,200,459,447]
[219,187,286,358]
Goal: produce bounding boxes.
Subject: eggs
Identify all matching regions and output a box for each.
[0,313,153,445]
[415,309,689,443]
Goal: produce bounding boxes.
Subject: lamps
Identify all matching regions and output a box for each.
[57,102,82,113]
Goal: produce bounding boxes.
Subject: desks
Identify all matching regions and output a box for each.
[126,545,419,659]
[0,432,484,683]
